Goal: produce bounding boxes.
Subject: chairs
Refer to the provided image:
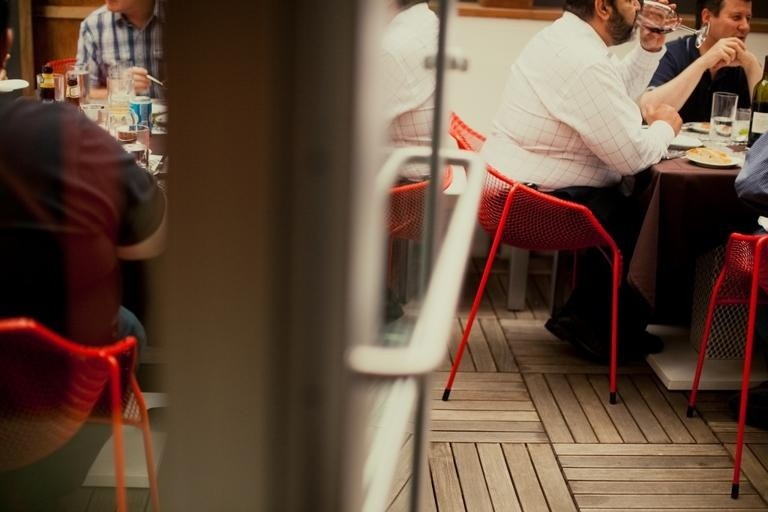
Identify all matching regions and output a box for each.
[387,164,453,291]
[0,315,159,512]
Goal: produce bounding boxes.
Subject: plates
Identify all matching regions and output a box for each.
[689,121,710,134]
[686,149,740,169]
[668,136,703,150]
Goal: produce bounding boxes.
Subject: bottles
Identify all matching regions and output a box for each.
[66,75,80,107]
[748,54,768,148]
[39,66,55,106]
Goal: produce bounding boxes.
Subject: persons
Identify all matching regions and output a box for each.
[71,1,169,106]
[1,1,171,511]
[731,124,767,431]
[638,1,768,125]
[474,0,687,369]
[372,0,441,325]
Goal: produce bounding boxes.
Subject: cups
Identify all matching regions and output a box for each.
[66,62,91,104]
[709,92,739,142]
[106,61,136,106]
[76,104,148,171]
[37,74,64,102]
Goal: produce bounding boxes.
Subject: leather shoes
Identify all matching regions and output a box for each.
[545,307,664,367]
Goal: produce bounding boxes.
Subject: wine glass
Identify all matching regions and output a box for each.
[636,0,714,50]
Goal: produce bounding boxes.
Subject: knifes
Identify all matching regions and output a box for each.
[153,153,168,176]
